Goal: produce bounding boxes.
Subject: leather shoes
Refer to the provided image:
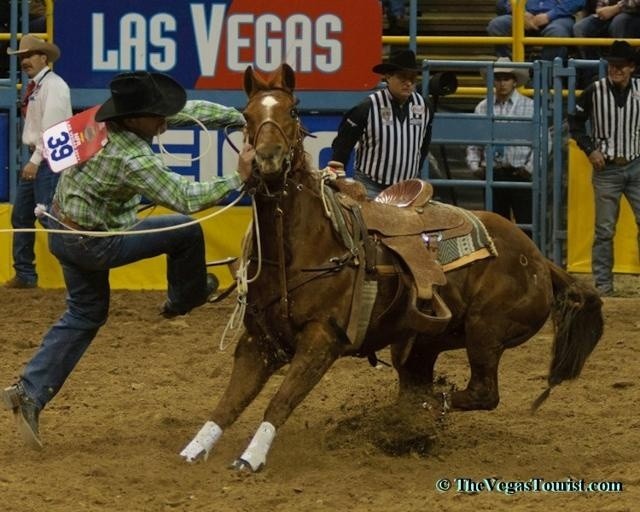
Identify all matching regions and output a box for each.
[5,383,50,455]
[158,272,222,320]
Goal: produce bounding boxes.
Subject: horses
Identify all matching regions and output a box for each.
[179,63,604,478]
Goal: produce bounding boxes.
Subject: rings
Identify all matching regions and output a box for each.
[29,176,32,179]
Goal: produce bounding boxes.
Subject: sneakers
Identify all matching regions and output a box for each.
[594,282,616,297]
[3,275,39,290]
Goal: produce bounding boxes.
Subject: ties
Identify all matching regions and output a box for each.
[20,81,35,118]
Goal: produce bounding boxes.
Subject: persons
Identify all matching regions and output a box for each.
[462,56,553,240]
[326,47,437,200]
[2,66,258,454]
[484,0,588,68]
[567,40,640,296]
[1,31,76,290]
[570,1,640,60]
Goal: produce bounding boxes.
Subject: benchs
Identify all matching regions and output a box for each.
[382,0,584,112]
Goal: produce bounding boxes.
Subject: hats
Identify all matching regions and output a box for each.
[601,39,640,65]
[371,49,432,75]
[94,68,187,126]
[6,32,62,63]
[479,56,531,87]
